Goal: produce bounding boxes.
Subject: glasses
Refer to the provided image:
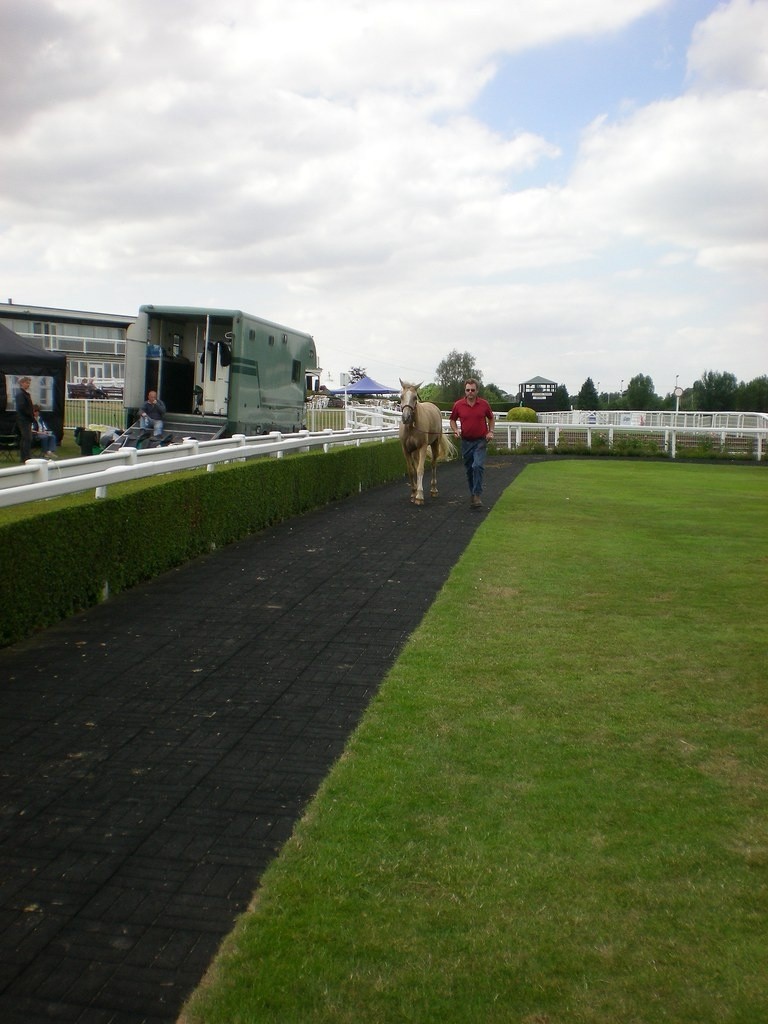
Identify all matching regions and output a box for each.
[466,389,475,392]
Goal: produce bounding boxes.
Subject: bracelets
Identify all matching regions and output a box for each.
[489,431,493,433]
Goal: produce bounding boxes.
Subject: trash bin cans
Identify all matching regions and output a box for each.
[79,430,101,456]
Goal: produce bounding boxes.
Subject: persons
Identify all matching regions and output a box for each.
[16,377,35,463]
[449,379,495,506]
[31,405,59,459]
[77,379,108,399]
[137,391,166,439]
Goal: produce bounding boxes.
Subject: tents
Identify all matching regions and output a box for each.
[0,322,67,449]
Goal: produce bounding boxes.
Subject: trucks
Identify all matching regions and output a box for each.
[101,305,322,455]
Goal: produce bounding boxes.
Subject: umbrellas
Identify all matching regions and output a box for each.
[328,376,401,409]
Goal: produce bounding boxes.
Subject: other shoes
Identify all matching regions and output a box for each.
[44,452,57,458]
[468,499,474,509]
[154,434,162,439]
[474,495,483,507]
[137,432,146,437]
[24,458,31,462]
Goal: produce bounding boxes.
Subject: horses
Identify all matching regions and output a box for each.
[398,377,460,506]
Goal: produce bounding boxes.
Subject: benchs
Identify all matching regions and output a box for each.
[101,386,123,399]
[67,385,89,396]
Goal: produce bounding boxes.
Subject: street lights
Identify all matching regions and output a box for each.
[620,379,624,395]
[597,381,600,397]
[676,374,680,386]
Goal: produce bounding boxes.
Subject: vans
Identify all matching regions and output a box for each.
[66,382,108,403]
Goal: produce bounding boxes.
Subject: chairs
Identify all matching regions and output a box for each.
[0,434,41,462]
